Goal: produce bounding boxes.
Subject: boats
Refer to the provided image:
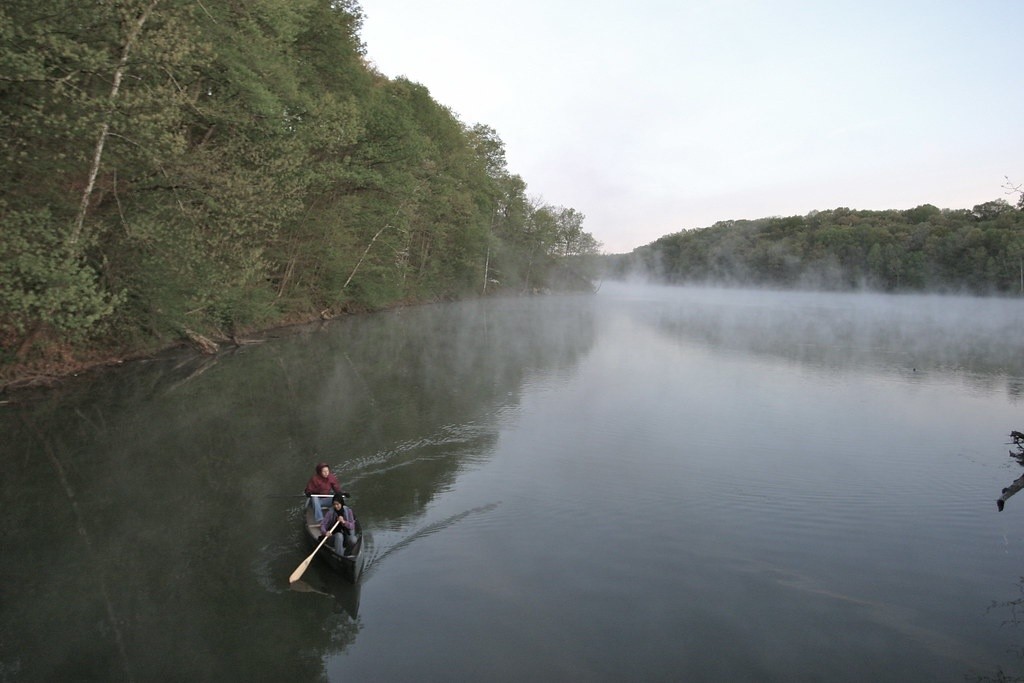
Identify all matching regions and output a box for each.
[306,497,366,584]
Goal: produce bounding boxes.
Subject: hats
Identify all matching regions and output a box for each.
[333,494,344,506]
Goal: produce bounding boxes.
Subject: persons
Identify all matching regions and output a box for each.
[305,463,350,524]
[321,494,357,556]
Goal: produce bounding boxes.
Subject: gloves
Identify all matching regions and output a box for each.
[342,492,350,498]
[306,493,312,498]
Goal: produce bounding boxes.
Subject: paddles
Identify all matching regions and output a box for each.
[260,492,346,499]
[288,519,341,584]
[290,579,336,599]
